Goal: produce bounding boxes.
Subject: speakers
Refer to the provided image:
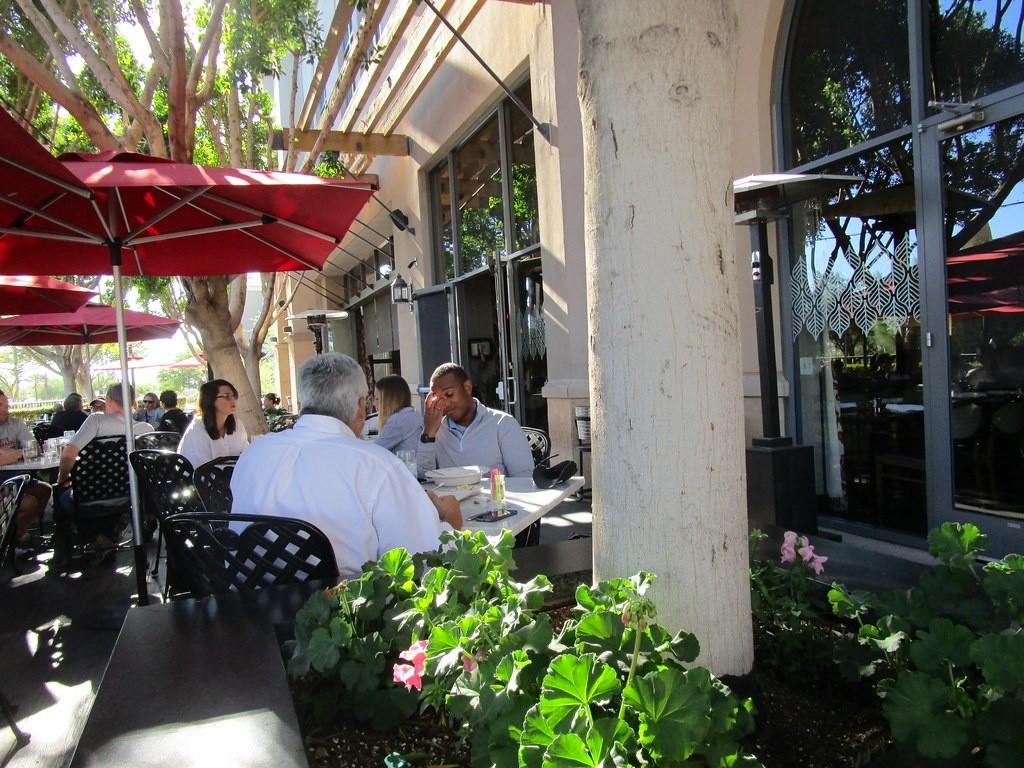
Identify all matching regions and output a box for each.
[390,209,409,231]
[279,301,287,310]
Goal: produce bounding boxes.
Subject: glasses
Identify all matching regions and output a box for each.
[143,399,155,405]
[215,391,236,401]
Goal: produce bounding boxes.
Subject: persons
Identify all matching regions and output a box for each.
[89,397,106,414]
[414,362,539,483]
[222,351,462,610]
[45,382,155,578]
[132,393,165,424]
[54,403,63,411]
[866,340,1024,454]
[177,379,251,481]
[47,393,89,439]
[154,389,190,442]
[371,374,424,463]
[0,389,51,549]
[263,392,281,408]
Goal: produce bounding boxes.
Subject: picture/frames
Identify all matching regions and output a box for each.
[469,338,491,357]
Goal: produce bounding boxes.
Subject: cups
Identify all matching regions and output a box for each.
[20,431,76,464]
[489,482,506,502]
[397,450,418,480]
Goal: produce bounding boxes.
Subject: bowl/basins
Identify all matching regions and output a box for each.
[425,465,490,485]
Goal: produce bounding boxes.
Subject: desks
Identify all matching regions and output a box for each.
[0,455,81,536]
[375,472,588,552]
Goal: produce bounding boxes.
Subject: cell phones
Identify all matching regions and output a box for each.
[475,508,518,522]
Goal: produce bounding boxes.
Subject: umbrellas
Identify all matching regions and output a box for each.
[837,230,1024,323]
[0,147,384,608]
[0,275,101,315]
[0,96,98,236]
[0,301,182,399]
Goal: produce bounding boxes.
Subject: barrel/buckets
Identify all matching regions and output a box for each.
[576,406,591,439]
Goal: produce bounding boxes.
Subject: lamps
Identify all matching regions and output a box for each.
[392,274,412,306]
[283,324,292,333]
[269,337,277,342]
[280,301,289,311]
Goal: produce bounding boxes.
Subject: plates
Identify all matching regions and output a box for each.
[435,486,483,501]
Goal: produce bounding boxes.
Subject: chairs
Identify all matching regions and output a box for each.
[573,405,591,503]
[0,407,551,598]
